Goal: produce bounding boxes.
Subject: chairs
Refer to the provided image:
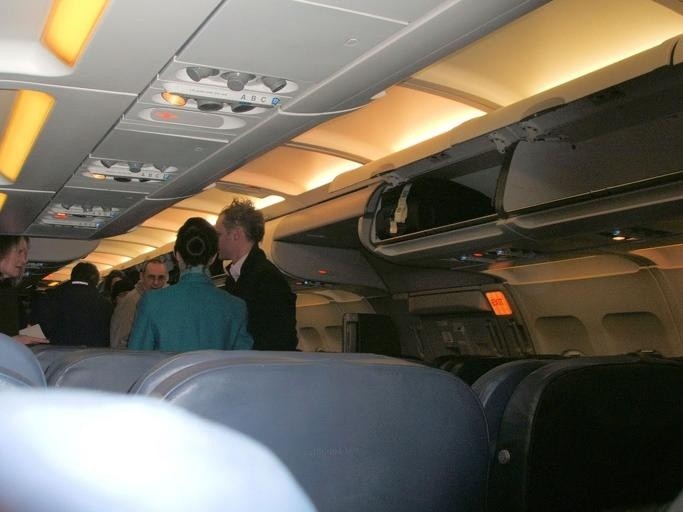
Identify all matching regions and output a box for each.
[0,334,490,511]
[408,353,682,512]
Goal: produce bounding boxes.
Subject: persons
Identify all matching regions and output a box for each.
[215,199,297,350]
[0,234,169,350]
[128,216,254,350]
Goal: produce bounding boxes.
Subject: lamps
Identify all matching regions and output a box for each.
[39,159,170,227]
[154,67,286,112]
